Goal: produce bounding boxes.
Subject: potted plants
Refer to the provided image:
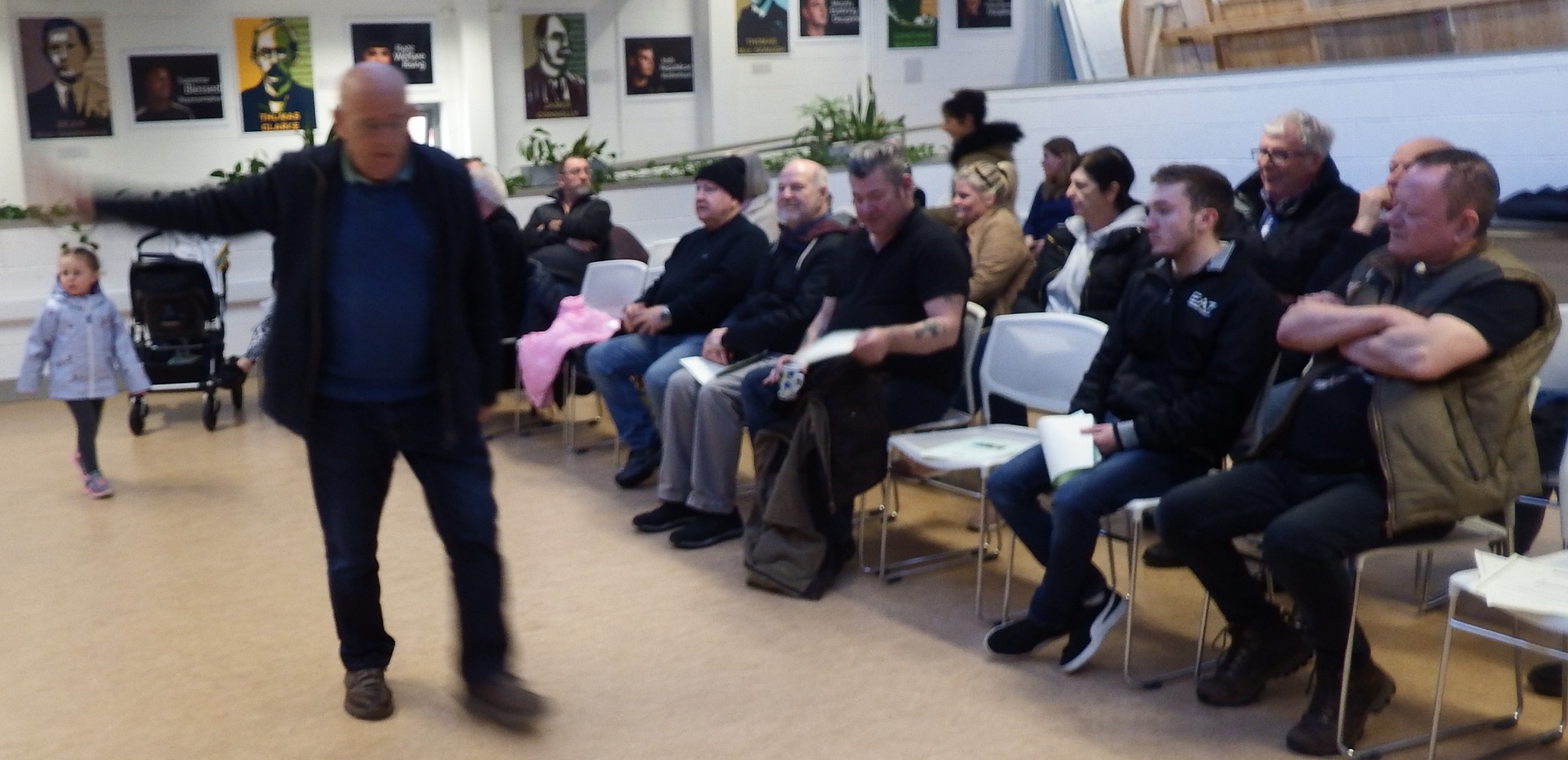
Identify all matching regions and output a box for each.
[789,74,908,163]
[515,127,618,188]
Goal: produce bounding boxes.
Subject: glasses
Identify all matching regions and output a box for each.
[1251,148,1306,162]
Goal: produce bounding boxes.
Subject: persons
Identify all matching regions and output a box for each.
[630,156,863,548]
[459,158,526,406]
[740,138,974,499]
[942,87,1020,226]
[34,61,555,729]
[981,164,1270,677]
[1006,146,1148,417]
[1230,109,1361,325]
[585,153,770,489]
[950,158,1037,324]
[1023,137,1080,296]
[1321,136,1452,294]
[1155,147,1560,755]
[520,154,613,426]
[16,244,150,499]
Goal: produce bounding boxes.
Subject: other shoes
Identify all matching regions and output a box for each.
[72,450,111,497]
[344,667,394,721]
[631,501,699,532]
[466,673,541,728]
[617,431,662,486]
[1528,660,1564,698]
[670,505,745,548]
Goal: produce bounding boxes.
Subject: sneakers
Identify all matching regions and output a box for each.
[1287,658,1395,755]
[1198,609,1312,704]
[1060,589,1127,674]
[983,619,1053,657]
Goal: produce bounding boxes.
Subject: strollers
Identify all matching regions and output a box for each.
[125,227,250,439]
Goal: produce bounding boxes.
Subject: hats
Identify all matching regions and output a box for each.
[695,155,747,203]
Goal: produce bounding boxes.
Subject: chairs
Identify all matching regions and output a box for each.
[511,259,668,469]
[854,299,994,576]
[876,312,1112,617]
[1336,512,1524,760]
[1192,531,1272,694]
[1112,492,1217,694]
[1427,443,1568,760]
